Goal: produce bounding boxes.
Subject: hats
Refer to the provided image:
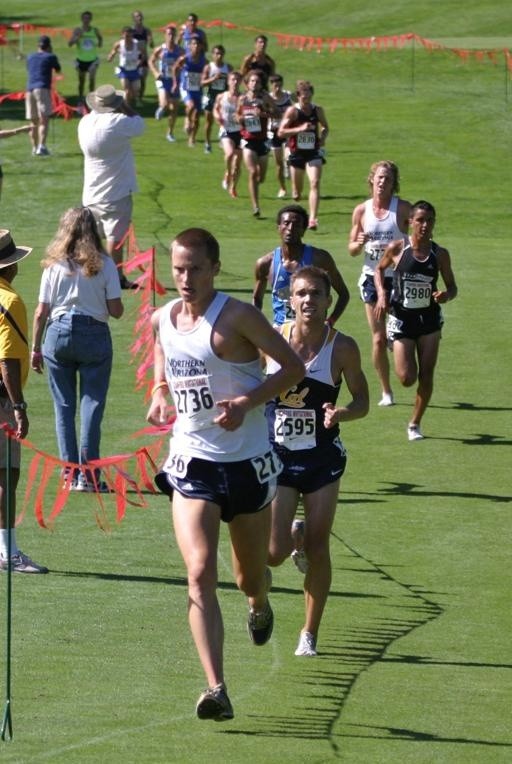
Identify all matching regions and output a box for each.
[85,84,127,114]
[0,228,33,269]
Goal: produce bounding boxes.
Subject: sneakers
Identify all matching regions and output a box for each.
[76,474,116,493]
[246,566,274,646]
[307,219,319,231]
[120,278,144,290]
[62,476,78,492]
[196,682,235,722]
[378,392,396,406]
[0,548,49,575]
[407,422,424,441]
[294,628,318,656]
[133,95,302,218]
[32,145,53,157]
[290,518,308,574]
